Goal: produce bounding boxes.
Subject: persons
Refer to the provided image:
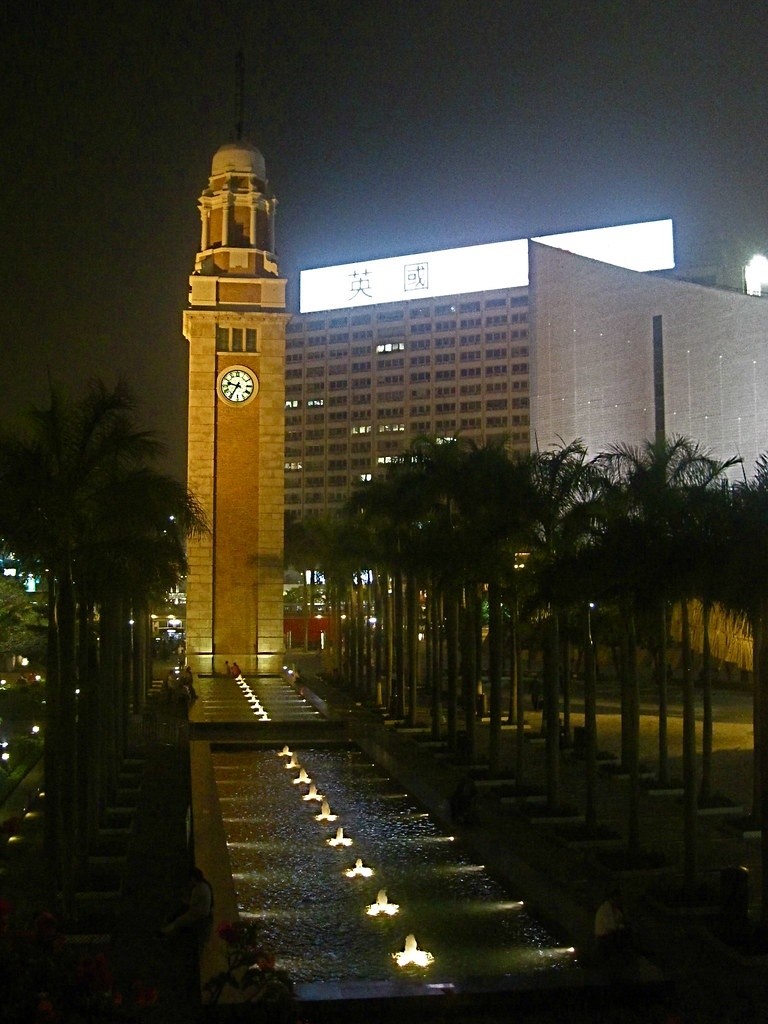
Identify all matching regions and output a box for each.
[449,775,477,826]
[164,870,214,953]
[225,661,241,675]
[528,676,542,711]
[16,674,28,684]
[178,644,185,671]
[176,683,191,706]
[595,889,629,950]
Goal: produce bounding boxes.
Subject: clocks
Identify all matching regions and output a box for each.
[216,364,259,408]
